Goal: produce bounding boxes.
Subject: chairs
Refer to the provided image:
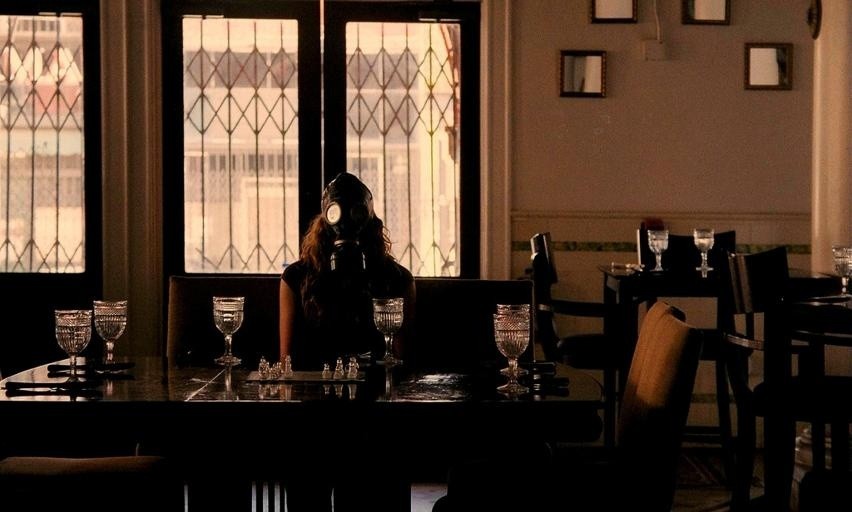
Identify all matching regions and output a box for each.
[0,450,169,510]
[430,227,852,511]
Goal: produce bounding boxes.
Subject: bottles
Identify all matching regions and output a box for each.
[258,383,357,399]
[258,356,359,381]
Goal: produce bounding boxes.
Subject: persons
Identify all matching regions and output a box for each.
[278,173,411,512]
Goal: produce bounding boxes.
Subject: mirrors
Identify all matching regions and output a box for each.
[590,1,732,28]
[558,48,606,100]
[741,41,796,91]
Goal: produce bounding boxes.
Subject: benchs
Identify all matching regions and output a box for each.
[164,270,537,372]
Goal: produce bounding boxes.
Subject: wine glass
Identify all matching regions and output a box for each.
[213,296,244,366]
[54,310,94,391]
[832,246,852,299]
[372,298,404,365]
[492,302,531,397]
[693,226,715,271]
[648,229,669,272]
[93,301,128,376]
